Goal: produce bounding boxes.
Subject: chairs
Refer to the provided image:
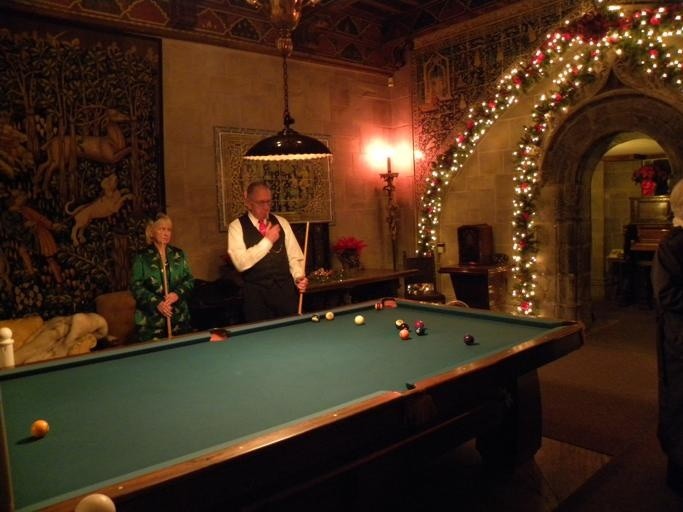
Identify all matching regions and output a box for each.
[401,247,446,305]
[93,290,136,346]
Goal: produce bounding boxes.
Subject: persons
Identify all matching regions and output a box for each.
[226,181,310,322]
[130,212,196,342]
[653,174,682,495]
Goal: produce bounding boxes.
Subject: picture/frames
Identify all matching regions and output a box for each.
[213,125,334,232]
[0,6,167,316]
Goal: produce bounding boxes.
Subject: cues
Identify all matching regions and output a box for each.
[236,407,483,512]
[298,222,309,315]
[161,219,172,339]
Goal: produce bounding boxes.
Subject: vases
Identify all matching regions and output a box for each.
[641,180,657,197]
[338,253,359,272]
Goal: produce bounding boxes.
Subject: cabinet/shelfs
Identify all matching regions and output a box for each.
[624,195,672,250]
[295,268,420,315]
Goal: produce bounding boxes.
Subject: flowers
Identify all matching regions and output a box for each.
[328,236,367,258]
[630,166,664,186]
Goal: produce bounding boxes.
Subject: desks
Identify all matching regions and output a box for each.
[0,298,587,511]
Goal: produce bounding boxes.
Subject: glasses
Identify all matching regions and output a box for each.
[249,198,272,206]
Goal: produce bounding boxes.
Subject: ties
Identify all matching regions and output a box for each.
[258,219,266,237]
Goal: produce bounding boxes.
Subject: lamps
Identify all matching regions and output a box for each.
[364,140,416,271]
[242,1,334,162]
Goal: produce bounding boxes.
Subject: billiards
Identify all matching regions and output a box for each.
[75,494,116,512]
[464,334,474,344]
[326,312,334,320]
[31,419,49,437]
[375,302,383,310]
[415,320,424,328]
[416,327,425,337]
[354,315,364,325]
[400,330,408,339]
[400,323,409,330]
[396,319,404,327]
